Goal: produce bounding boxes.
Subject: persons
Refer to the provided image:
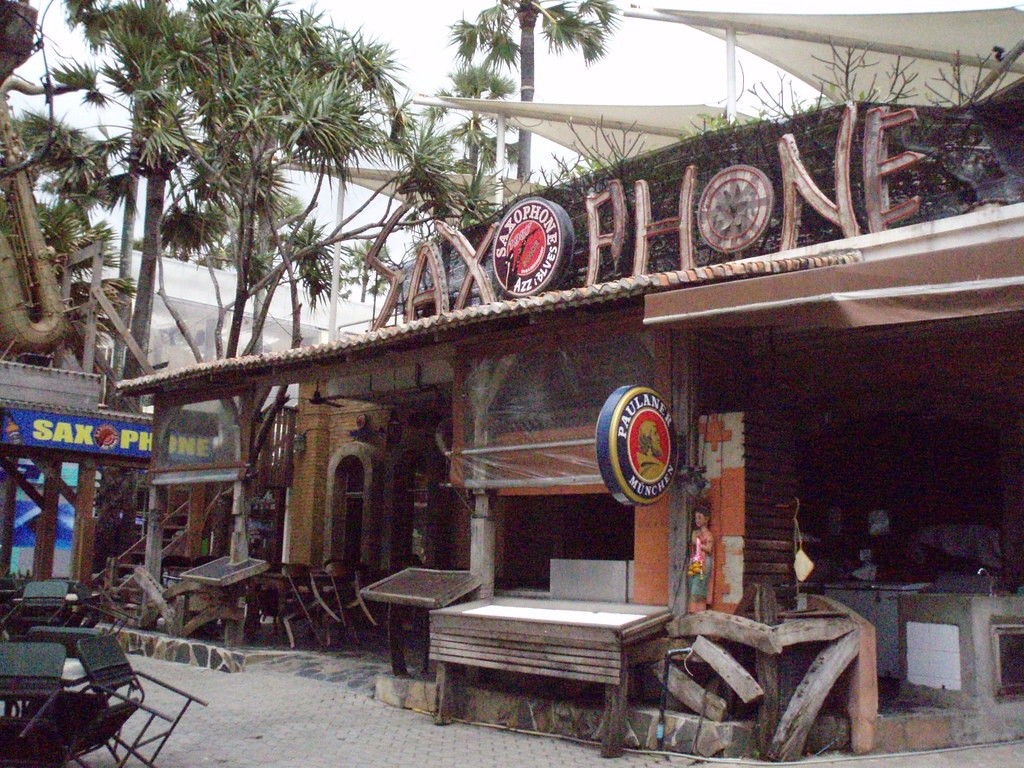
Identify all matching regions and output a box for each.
[688,501,713,614]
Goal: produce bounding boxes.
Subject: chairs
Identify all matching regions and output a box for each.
[0,557,379,768]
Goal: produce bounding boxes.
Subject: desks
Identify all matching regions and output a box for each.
[428,595,674,758]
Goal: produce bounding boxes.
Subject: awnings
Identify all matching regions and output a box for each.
[642,236,1024,345]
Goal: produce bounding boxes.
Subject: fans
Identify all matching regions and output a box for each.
[289,381,346,408]
